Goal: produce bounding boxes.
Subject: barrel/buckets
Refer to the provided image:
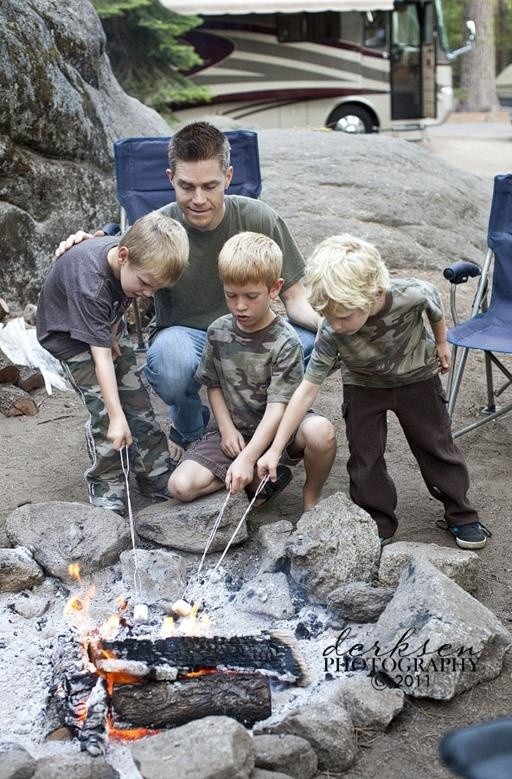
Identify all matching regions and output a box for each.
[248,465,294,510]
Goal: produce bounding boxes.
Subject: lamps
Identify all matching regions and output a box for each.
[114,131,261,350]
[442,174,511,441]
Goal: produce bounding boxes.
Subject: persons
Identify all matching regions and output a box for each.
[167,231,338,515]
[54,120,342,448]
[35,215,191,516]
[258,232,486,549]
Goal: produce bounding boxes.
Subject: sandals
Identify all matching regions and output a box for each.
[160,0,478,136]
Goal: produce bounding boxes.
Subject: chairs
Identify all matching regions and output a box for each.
[453,522,487,550]
